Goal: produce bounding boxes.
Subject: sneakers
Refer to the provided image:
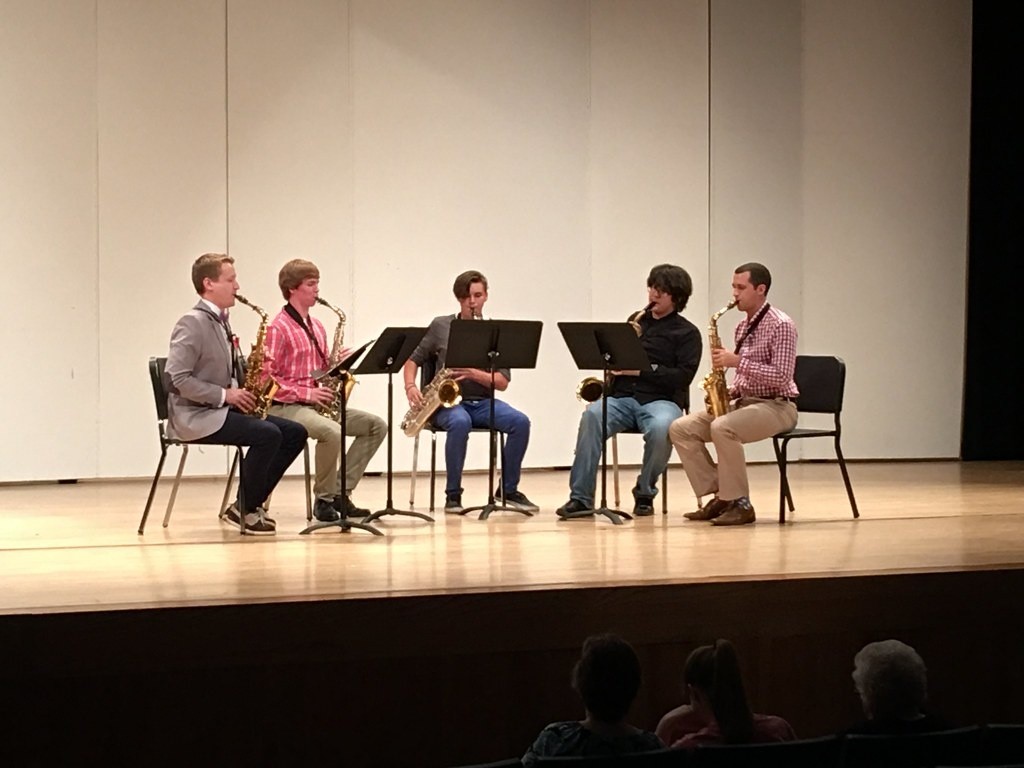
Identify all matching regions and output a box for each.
[222,504,276,535]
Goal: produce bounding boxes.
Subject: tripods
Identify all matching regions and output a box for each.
[557,322,658,524]
[300,319,544,536]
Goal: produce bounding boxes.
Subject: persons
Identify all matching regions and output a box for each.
[523,632,950,768]
[257,259,389,520]
[557,264,702,516]
[669,263,800,525]
[404,271,539,513]
[162,252,308,535]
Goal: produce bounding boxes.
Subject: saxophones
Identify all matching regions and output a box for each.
[575,301,657,406]
[697,299,740,420]
[234,292,286,423]
[400,307,483,438]
[314,295,361,421]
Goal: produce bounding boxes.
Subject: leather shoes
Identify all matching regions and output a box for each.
[710,497,755,525]
[334,495,370,517]
[313,499,339,521]
[683,497,732,520]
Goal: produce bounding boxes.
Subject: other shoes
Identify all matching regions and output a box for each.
[556,499,593,518]
[444,488,464,512]
[635,499,653,515]
[494,486,539,512]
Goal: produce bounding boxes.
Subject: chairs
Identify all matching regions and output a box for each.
[409,361,506,512]
[138,356,313,534]
[772,355,859,523]
[458,724,1024,768]
[611,386,702,514]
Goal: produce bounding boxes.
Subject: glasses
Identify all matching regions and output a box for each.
[647,286,672,295]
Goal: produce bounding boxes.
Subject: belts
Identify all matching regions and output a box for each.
[759,396,795,401]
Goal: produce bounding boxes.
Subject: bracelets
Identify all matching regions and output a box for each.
[405,383,416,393]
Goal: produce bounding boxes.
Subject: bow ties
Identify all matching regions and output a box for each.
[218,309,230,322]
